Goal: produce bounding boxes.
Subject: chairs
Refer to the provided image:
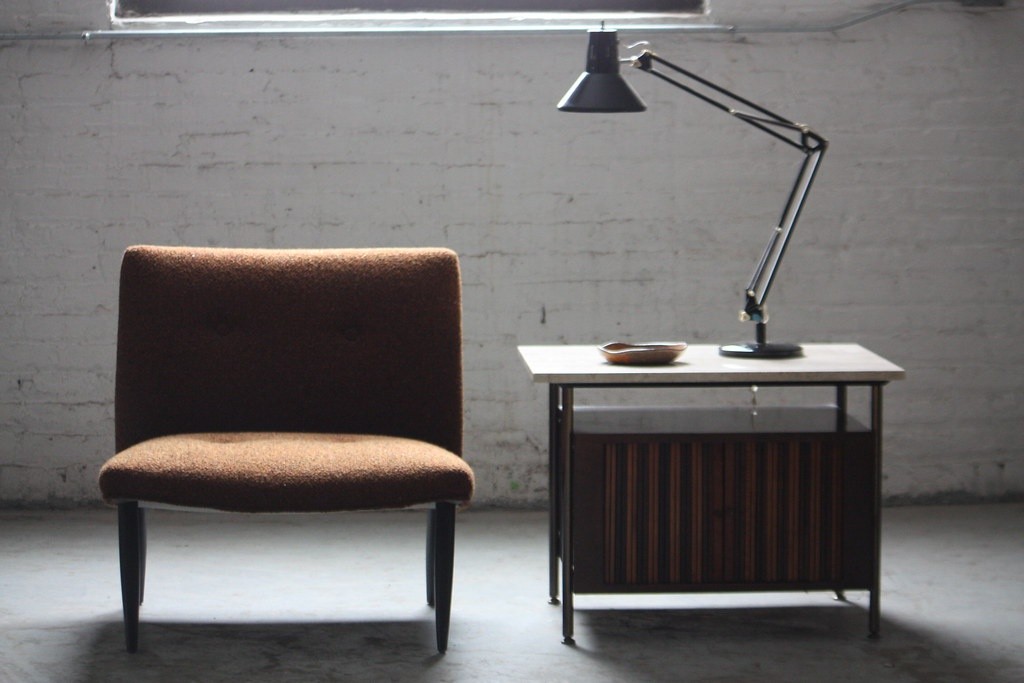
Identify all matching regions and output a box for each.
[99,244,474,652]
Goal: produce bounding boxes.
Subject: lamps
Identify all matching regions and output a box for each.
[557,20,828,357]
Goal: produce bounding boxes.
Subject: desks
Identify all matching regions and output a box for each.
[517,343,906,645]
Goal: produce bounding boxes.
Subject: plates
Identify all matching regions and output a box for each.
[598,342,687,364]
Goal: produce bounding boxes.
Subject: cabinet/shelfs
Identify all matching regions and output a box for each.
[559,406,872,593]
